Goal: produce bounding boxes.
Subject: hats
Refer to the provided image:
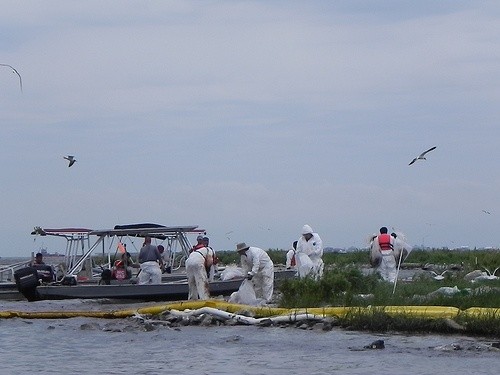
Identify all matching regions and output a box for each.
[197,235,203,239]
[236,242,249,252]
[302,225,313,235]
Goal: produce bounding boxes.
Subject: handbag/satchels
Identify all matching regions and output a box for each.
[238,278,256,303]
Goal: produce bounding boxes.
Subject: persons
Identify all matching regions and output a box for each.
[29,253,44,266]
[234,242,274,304]
[286,241,298,270]
[295,225,324,281]
[112,252,140,280]
[137,237,164,284]
[370,226,398,283]
[185,235,218,300]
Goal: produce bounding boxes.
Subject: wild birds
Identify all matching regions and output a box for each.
[421,266,448,281]
[480,209,490,215]
[0,63,23,94]
[62,154,78,168]
[447,260,465,272]
[476,262,500,280]
[408,146,437,166]
[131,306,172,326]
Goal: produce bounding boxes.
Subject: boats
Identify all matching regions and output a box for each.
[0,223,297,303]
